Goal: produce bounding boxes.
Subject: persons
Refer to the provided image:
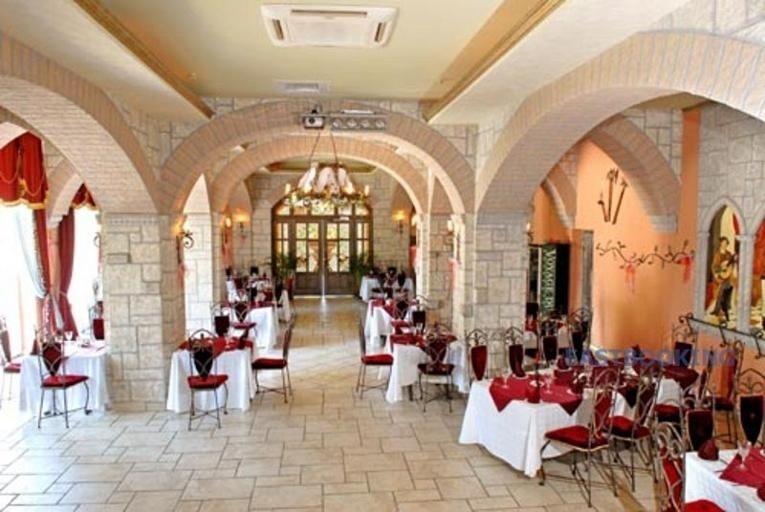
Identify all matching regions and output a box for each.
[708,233,735,322]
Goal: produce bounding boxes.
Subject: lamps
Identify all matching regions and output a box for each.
[282,118,371,213]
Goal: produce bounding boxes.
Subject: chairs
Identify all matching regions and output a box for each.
[456,307,764,511]
[0,300,105,429]
[186,266,295,432]
[355,267,457,415]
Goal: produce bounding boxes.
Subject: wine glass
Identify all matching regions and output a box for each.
[416,323,423,337]
[501,366,512,389]
[736,439,751,472]
[544,371,554,395]
[224,333,233,349]
[584,363,593,388]
[65,330,73,352]
